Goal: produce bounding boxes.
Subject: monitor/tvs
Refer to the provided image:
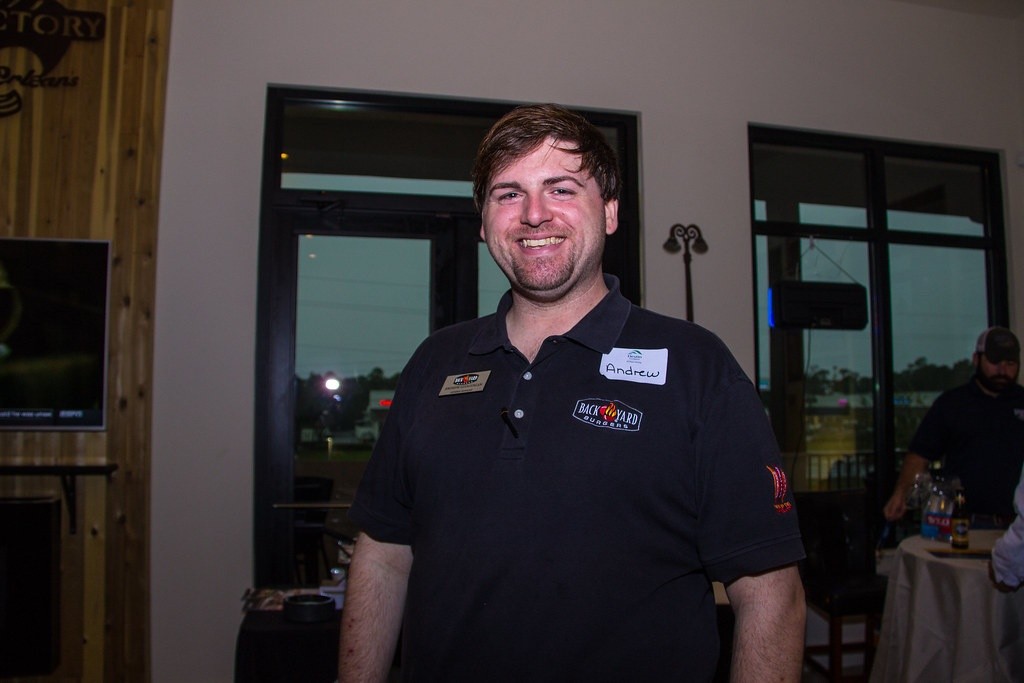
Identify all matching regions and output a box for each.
[0,237,112,431]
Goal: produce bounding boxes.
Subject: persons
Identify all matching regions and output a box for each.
[884,326,1024,529]
[988,465,1024,592]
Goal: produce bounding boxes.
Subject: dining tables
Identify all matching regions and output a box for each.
[867,530,1024,683]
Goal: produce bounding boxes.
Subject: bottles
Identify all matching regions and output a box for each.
[923,488,969,549]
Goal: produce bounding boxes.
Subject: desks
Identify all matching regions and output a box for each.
[233,610,342,683]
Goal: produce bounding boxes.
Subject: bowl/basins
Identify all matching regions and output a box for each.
[283,595,335,622]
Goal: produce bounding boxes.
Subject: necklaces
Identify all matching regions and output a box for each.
[338,104,808,683]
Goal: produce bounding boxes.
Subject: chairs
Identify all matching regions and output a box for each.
[794,488,886,683]
